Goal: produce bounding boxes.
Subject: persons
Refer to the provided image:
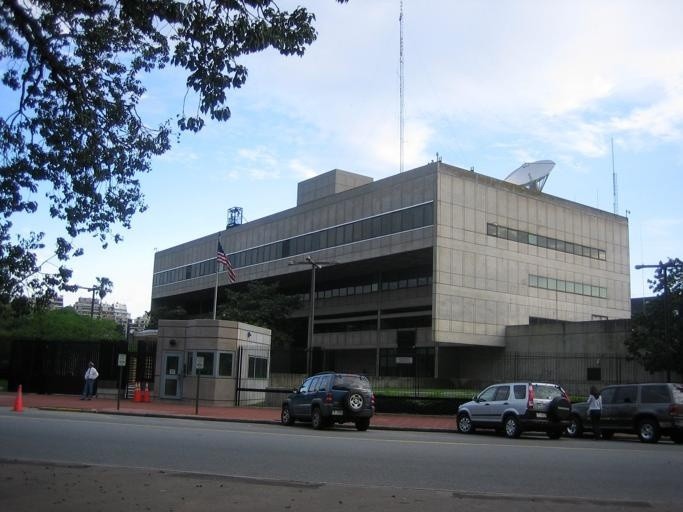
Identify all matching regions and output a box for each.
[80,361,98,400]
[587,387,604,441]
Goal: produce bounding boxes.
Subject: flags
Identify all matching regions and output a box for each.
[216,240,236,285]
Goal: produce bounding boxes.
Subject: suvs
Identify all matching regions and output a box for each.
[564,381,683,445]
[279,371,376,433]
[455,383,572,441]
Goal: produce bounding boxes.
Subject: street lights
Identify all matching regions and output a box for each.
[635,261,682,381]
[71,283,111,323]
[288,256,333,379]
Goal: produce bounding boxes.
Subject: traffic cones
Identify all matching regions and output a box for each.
[11,384,24,412]
[133,383,151,403]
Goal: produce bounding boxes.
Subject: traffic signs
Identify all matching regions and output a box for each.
[118,354,126,366]
